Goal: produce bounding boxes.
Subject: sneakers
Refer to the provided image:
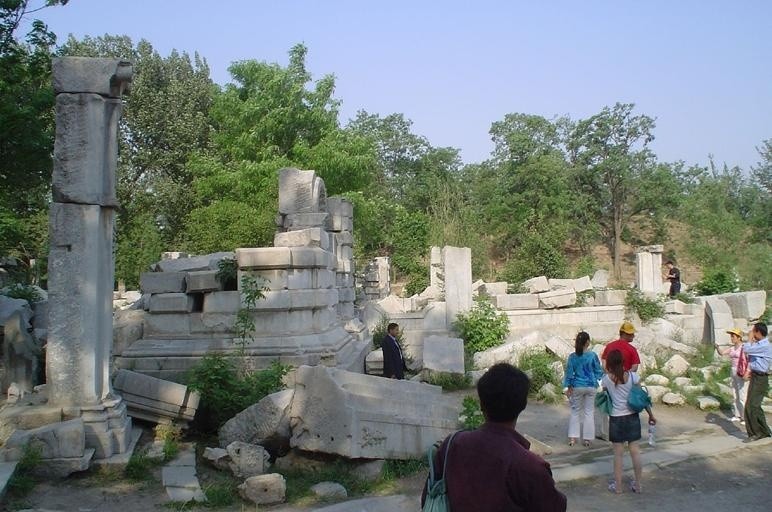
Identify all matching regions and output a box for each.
[742,431,771,445]
[727,415,747,428]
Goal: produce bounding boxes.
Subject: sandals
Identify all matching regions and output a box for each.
[607,479,643,496]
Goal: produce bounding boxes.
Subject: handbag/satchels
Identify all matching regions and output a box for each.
[736,345,748,377]
[420,429,465,511]
[626,372,652,413]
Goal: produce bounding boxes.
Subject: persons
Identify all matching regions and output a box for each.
[601,320,642,377]
[422,361,568,511]
[712,326,750,426]
[741,321,772,443]
[381,321,409,380]
[594,349,658,496]
[660,261,681,298]
[562,330,606,447]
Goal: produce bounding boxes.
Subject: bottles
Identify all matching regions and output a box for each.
[568,394,577,416]
[647,423,655,446]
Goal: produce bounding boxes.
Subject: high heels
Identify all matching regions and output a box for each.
[567,439,592,450]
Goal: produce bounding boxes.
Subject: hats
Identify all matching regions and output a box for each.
[725,328,744,338]
[618,321,638,335]
